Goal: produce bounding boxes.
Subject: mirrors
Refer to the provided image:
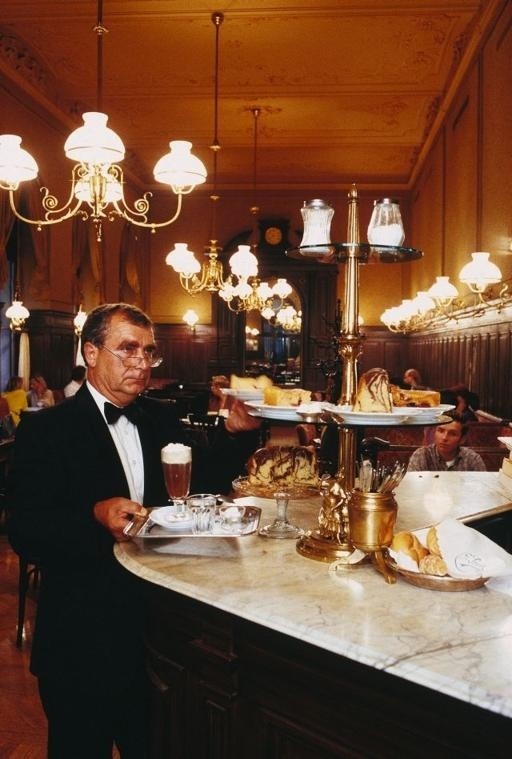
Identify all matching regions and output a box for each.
[237,276,308,389]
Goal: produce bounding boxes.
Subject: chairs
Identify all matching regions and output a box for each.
[9,519,46,648]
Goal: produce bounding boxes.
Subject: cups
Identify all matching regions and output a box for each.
[187,494,216,533]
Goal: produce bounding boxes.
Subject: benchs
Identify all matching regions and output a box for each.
[363,421,512,444]
[360,437,509,471]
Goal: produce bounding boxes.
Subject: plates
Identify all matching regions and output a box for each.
[149,506,193,530]
[321,406,410,422]
[394,403,456,417]
[383,548,489,593]
[243,400,298,412]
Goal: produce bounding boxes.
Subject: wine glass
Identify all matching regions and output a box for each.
[161,449,192,522]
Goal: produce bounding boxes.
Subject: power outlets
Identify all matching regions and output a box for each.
[219,203,293,312]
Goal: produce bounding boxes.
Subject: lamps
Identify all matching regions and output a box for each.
[72,302,88,336]
[379,253,509,335]
[182,308,199,331]
[259,305,303,336]
[0,1,207,242]
[5,291,30,334]
[245,325,260,351]
[165,14,258,296]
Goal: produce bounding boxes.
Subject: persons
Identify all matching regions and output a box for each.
[403,368,486,472]
[1,366,232,427]
[0,303,261,759]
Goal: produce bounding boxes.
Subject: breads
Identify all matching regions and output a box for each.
[394,390,441,407]
[426,526,441,555]
[264,386,313,407]
[418,554,448,576]
[351,367,392,413]
[392,531,429,563]
[246,446,321,486]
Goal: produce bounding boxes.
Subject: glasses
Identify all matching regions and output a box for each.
[97,342,164,369]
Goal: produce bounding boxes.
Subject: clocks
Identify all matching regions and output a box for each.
[264,226,284,246]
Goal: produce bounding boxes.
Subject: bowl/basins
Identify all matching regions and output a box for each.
[219,505,245,524]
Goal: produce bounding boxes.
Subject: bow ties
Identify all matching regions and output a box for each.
[102,401,141,426]
